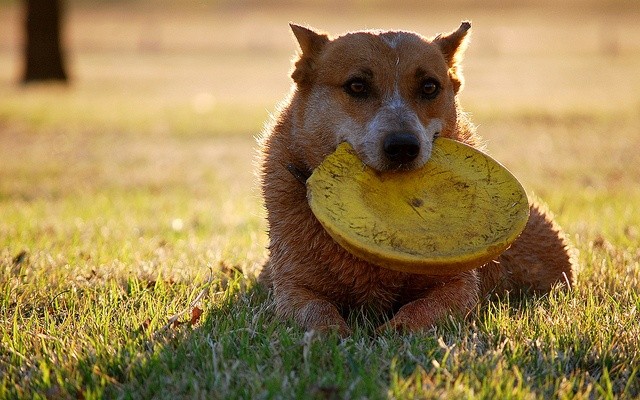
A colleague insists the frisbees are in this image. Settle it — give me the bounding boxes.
[306,134,531,275]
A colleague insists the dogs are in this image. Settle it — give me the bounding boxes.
[251,18,576,347]
